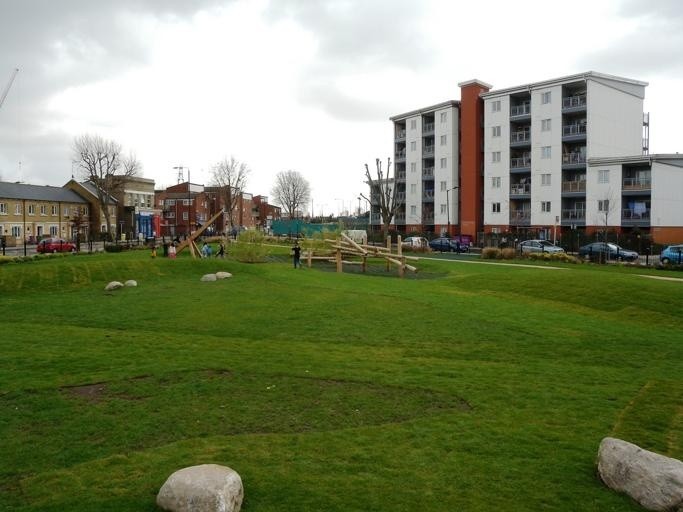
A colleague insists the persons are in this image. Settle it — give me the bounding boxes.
[162,231,191,266]
[455,240,460,255]
[196,228,239,260]
[150,247,157,258]
[291,244,302,270]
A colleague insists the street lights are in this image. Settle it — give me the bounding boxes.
[447,186,461,238]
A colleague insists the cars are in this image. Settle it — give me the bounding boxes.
[660,244,683,264]
[579,241,639,262]
[517,240,564,254]
[401,237,469,254]
[37,238,77,253]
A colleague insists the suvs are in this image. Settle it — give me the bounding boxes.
[228,225,247,236]
